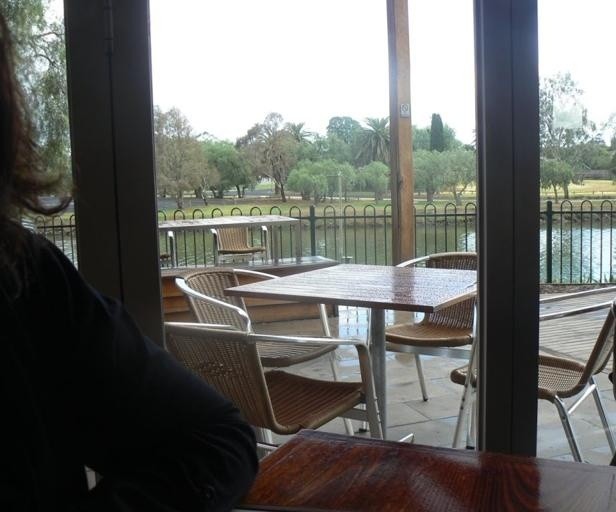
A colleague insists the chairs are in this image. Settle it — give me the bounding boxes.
[174,267,355,435]
[163,322,384,459]
[158,226,269,266]
[368,253,478,402]
[450,286,616,463]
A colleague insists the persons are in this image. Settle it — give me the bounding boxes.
[0,4,260,512]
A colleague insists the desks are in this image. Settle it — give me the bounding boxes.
[234,426,614,510]
[157,215,302,267]
